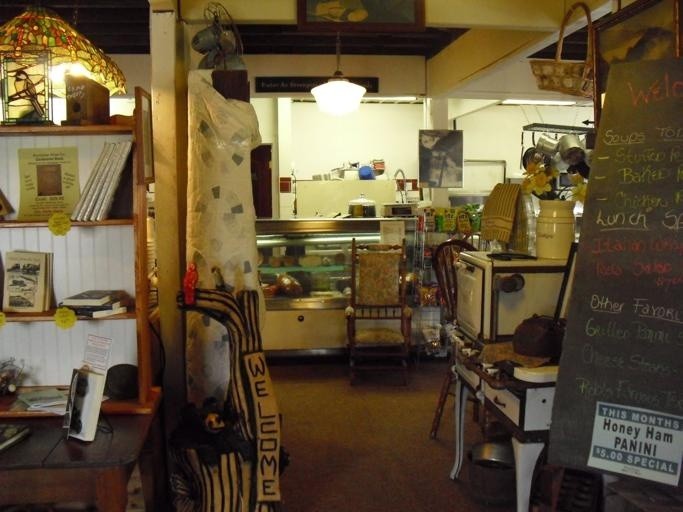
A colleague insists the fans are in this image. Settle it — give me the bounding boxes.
[190,1,244,69]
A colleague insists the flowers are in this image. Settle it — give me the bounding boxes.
[520,152,590,206]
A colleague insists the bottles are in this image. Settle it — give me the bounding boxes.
[279,177,291,194]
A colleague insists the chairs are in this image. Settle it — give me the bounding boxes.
[430,238,480,440]
[345,238,414,388]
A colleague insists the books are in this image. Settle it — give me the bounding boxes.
[11,396,70,416]
[3,138,140,320]
[15,386,68,408]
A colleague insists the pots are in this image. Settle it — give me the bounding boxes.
[347,193,376,219]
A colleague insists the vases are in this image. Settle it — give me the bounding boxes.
[534,200,574,260]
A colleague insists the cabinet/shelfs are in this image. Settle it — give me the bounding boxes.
[255,217,425,359]
[0,85,160,415]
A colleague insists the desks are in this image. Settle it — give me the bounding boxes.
[0,414,165,512]
[445,324,608,512]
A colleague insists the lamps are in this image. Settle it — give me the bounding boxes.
[311,32,366,117]
[0,0,126,98]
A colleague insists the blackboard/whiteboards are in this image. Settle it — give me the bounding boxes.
[545,58,681,493]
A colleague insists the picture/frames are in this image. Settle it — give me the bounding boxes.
[0,51,52,126]
[134,85,156,186]
[296,0,426,34]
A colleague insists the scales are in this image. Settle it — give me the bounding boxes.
[380,201,421,217]
